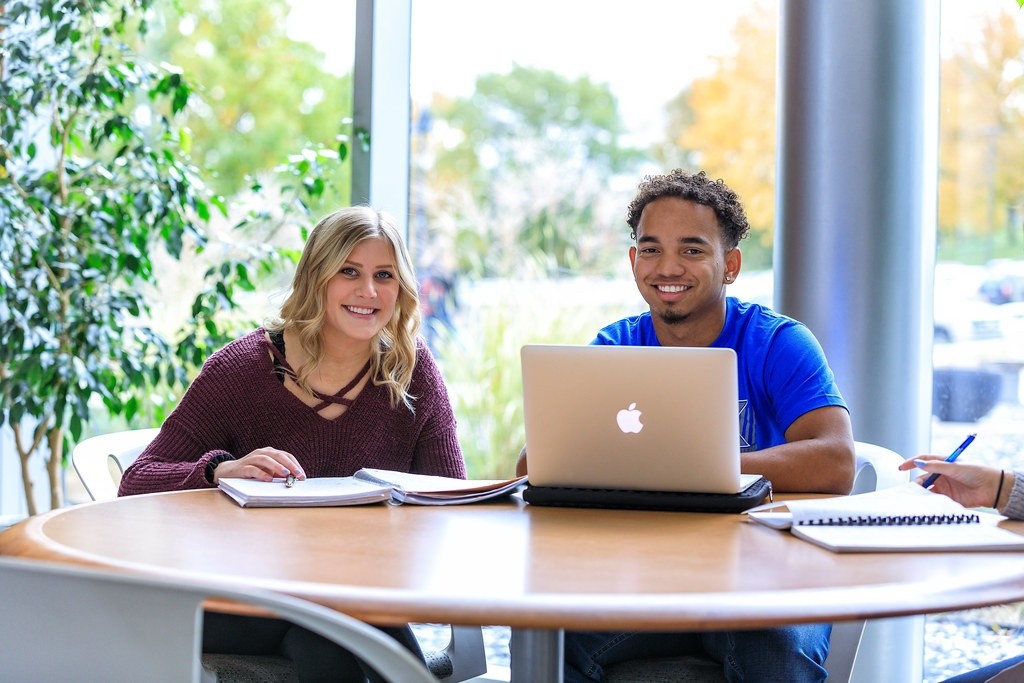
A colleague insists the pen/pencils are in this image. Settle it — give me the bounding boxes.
[286,474,296,487]
[920,430,977,488]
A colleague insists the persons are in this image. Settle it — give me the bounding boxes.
[901,453,1022,682]
[114,203,465,683]
[514,167,860,683]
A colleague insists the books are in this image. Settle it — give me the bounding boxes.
[218,468,529,511]
[742,477,1020,551]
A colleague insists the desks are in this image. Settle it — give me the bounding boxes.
[0,491,1024,683]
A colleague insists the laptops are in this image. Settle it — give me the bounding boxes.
[520,343,764,495]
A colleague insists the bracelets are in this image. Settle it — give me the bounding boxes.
[993,470,1005,509]
[205,451,235,483]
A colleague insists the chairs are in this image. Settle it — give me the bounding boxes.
[0,556,444,683]
[72,428,486,683]
[807,435,908,683]
